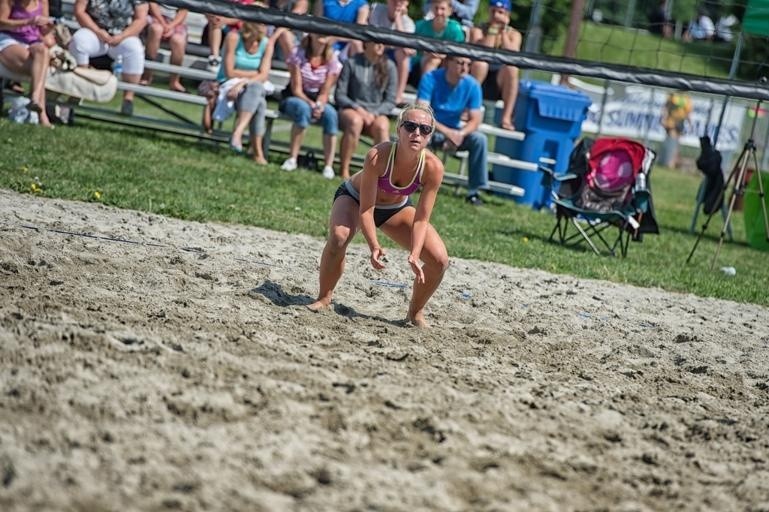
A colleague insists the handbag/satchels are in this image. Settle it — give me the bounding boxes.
[573,176,629,215]
[49,50,76,75]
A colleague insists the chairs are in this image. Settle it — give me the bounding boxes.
[547,136,656,259]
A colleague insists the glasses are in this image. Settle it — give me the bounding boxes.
[453,58,472,66]
[400,120,432,135]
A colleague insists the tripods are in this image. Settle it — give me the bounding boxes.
[687,100,769,272]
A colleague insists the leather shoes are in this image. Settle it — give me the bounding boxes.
[228,136,242,153]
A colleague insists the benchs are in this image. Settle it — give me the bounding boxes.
[0,0,537,198]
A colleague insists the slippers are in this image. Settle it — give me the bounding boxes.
[500,120,515,132]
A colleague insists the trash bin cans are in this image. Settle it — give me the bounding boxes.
[485,79,593,211]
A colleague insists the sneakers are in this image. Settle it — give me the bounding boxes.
[122,100,134,116]
[322,165,335,180]
[465,193,483,206]
[206,54,222,74]
[280,158,297,171]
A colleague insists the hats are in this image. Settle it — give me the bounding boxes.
[489,1,511,10]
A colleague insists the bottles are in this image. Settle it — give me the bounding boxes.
[113,56,124,80]
[635,172,646,190]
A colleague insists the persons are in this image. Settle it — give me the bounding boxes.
[197,0,523,206]
[0,0,190,127]
[583,1,740,50]
[303,105,449,327]
